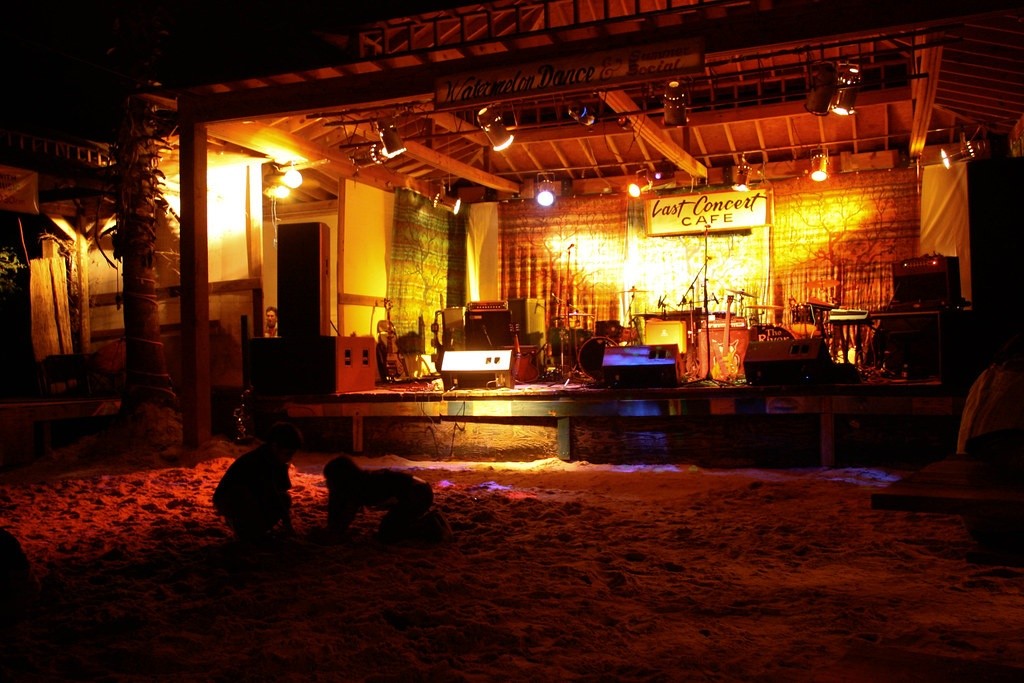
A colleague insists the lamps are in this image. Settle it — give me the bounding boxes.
[840,151,857,174]
[349,125,407,169]
[478,101,514,150]
[569,99,595,126]
[808,147,830,182]
[653,160,674,180]
[661,79,691,132]
[561,176,573,198]
[940,139,983,169]
[537,173,556,207]
[627,168,654,197]
[803,62,863,117]
[263,170,303,199]
[732,153,751,191]
[433,192,461,215]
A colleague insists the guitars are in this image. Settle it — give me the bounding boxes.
[384,298,405,378]
[711,295,738,381]
[509,321,539,383]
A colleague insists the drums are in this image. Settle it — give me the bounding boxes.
[750,304,818,341]
[548,320,638,383]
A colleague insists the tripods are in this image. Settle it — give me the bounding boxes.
[680,225,736,387]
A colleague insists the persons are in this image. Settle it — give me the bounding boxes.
[264,307,278,336]
[323,455,455,544]
[213,423,304,548]
[955,332,1024,454]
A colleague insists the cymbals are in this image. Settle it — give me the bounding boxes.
[725,289,758,298]
[744,305,785,310]
[804,280,840,288]
[558,311,593,316]
[619,289,650,293]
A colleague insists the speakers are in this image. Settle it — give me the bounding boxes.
[247,336,376,395]
[441,350,515,391]
[509,298,547,366]
[277,222,331,337]
[602,344,684,388]
[743,338,832,386]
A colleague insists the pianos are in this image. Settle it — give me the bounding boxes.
[829,308,869,367]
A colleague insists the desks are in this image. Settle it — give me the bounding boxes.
[872,462,1024,546]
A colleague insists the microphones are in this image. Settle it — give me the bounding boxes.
[567,243,574,249]
